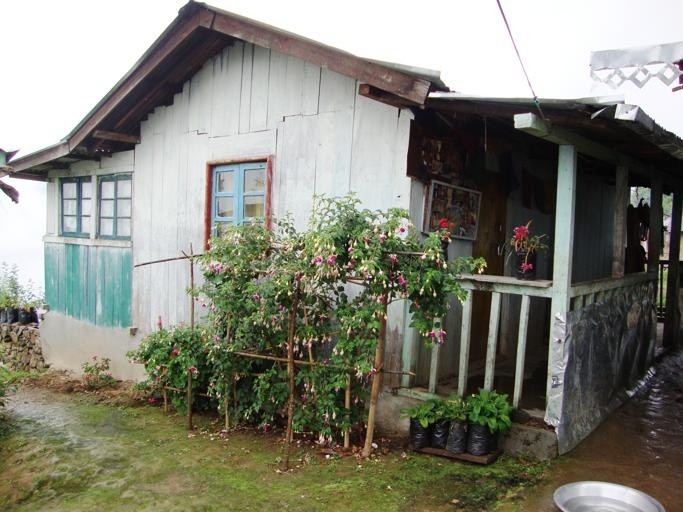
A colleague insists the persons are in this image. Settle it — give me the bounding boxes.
[624,232,647,274]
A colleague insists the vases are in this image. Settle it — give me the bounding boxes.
[517,266,537,280]
[410,416,489,454]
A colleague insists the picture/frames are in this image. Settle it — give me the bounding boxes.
[420,180,483,241]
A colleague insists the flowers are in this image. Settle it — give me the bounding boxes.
[498,217,552,278]
[400,387,515,436]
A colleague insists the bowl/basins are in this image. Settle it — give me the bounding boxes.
[553,480,665,512]
